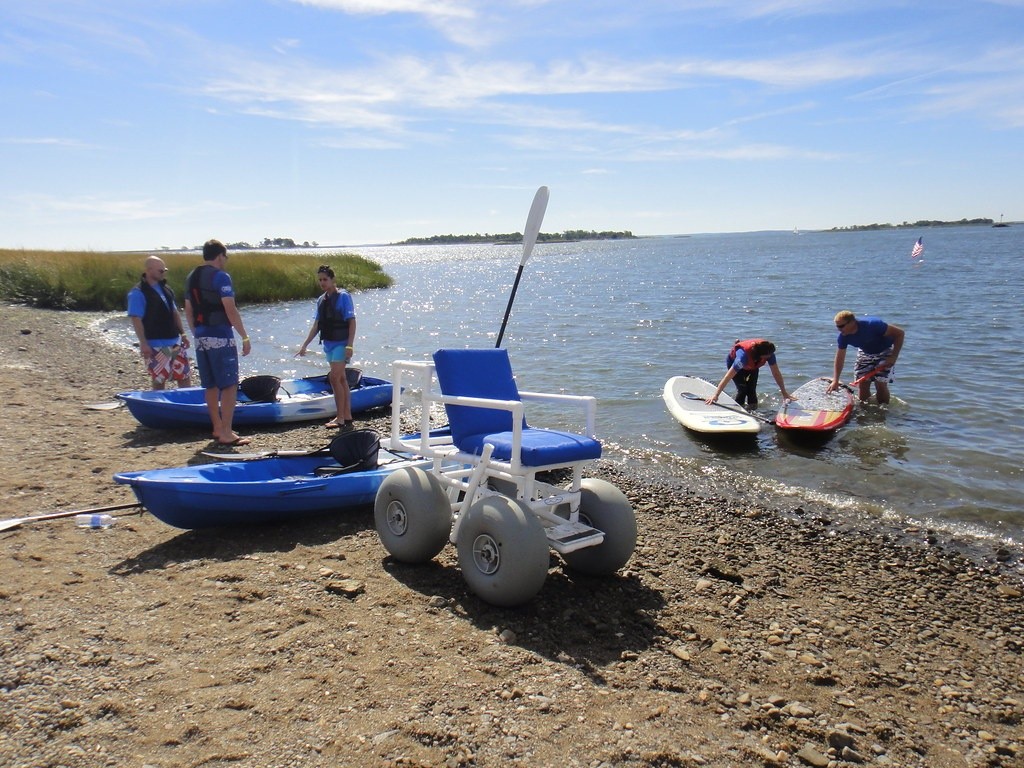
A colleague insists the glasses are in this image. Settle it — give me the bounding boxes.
[318,278,330,282]
[154,267,169,274]
[223,253,229,261]
[836,320,852,329]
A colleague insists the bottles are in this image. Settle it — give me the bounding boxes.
[74,515,117,527]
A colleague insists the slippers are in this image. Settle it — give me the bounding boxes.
[212,428,249,447]
[325,418,354,429]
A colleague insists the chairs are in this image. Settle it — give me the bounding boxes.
[432,347,602,469]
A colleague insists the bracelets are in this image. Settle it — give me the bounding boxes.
[345,345,353,349]
[182,333,186,337]
[242,335,249,341]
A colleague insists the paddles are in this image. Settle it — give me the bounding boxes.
[681,393,776,425]
[0,501,143,530]
[82,401,126,411]
[495,185,550,349]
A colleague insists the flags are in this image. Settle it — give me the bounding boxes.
[912,238,921,256]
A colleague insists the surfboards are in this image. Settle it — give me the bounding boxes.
[663,375,761,433]
[775,377,853,431]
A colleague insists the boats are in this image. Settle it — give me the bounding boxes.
[112,424,478,529]
[118,374,405,430]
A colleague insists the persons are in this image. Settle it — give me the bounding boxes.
[293,266,355,427]
[704,339,798,404]
[825,310,905,403]
[126,256,191,390]
[183,240,251,445]
